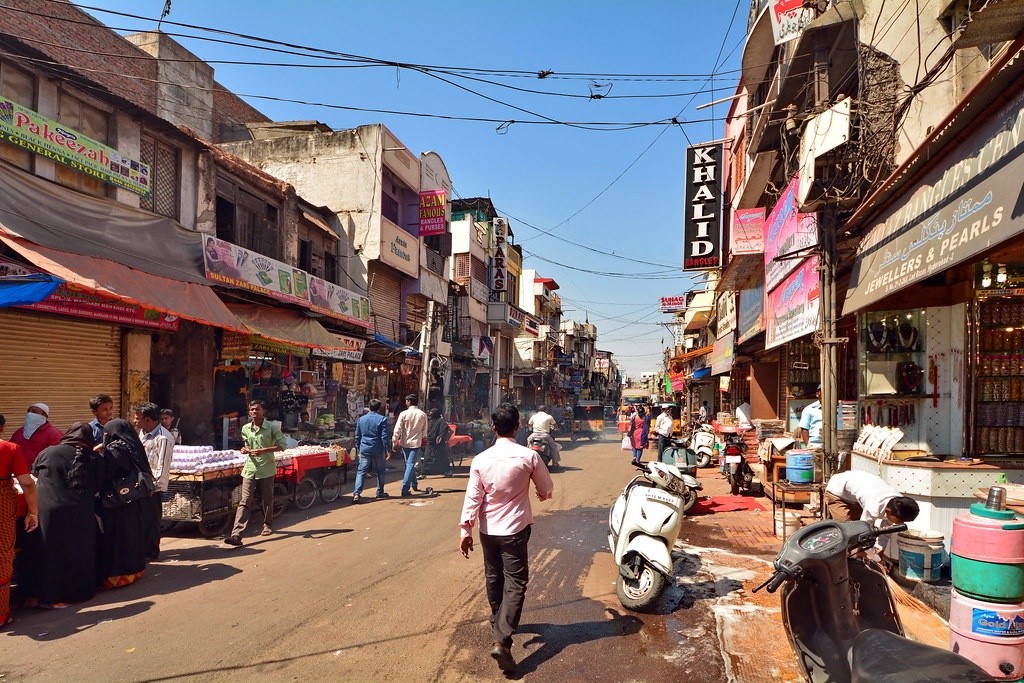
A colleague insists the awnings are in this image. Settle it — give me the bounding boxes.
[222,292,356,350]
[670,346,714,360]
[0,233,251,343]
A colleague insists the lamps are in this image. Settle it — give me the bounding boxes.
[374,366,378,372]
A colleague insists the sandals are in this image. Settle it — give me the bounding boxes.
[0,615,15,631]
[262,526,273,536]
[224,536,243,546]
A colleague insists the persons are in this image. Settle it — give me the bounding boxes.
[824,470,920,572]
[353,394,454,501]
[224,400,287,546]
[516,401,573,468]
[626,397,752,469]
[0,393,182,624]
[458,403,553,672]
[799,383,823,448]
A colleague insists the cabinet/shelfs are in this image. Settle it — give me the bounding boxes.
[866,310,934,400]
[788,342,841,398]
[970,266,1024,456]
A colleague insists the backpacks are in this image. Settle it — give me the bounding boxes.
[65,446,95,502]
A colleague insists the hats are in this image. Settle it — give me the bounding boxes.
[661,405,669,408]
[31,403,50,416]
[817,383,821,389]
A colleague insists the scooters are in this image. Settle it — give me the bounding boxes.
[751,521,998,683]
[607,455,706,612]
[644,413,756,515]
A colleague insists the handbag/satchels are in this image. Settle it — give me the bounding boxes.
[259,350,273,382]
[622,435,632,451]
[99,445,139,508]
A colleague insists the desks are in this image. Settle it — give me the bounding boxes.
[397,426,472,470]
[771,481,825,545]
[165,459,296,536]
[763,456,811,503]
[273,446,349,507]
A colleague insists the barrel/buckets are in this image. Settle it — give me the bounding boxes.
[786,453,813,484]
[774,512,801,542]
[474,440,484,452]
[896,530,945,582]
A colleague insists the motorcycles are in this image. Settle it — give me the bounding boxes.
[526,422,560,468]
[552,388,683,444]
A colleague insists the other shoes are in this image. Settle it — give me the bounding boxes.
[418,475,425,480]
[551,463,564,472]
[402,492,417,498]
[413,487,421,491]
[445,472,452,478]
[52,602,78,609]
[491,641,516,671]
[352,495,360,504]
[375,493,389,500]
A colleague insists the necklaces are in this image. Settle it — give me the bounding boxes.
[897,325,915,348]
[868,324,888,349]
